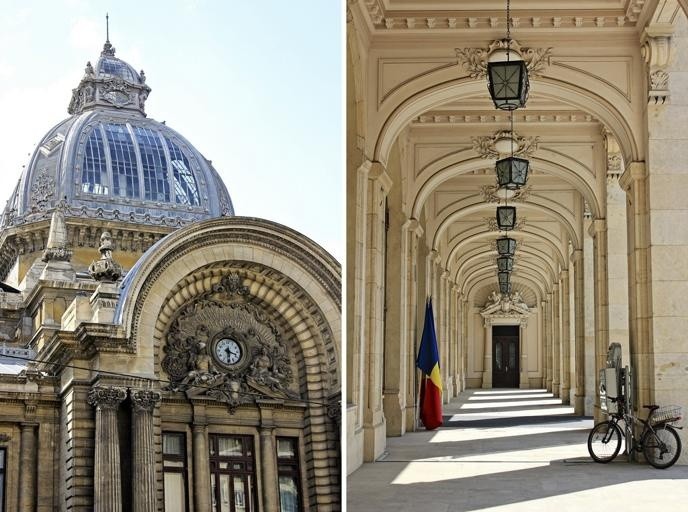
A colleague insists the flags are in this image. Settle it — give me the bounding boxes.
[415,294,444,432]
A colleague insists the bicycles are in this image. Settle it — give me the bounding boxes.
[586,394,684,470]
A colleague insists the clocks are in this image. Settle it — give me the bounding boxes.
[210,331,247,372]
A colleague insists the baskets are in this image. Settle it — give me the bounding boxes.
[651,405,682,424]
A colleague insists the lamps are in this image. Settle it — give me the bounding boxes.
[495,110,529,190]
[485,0,529,111]
[495,190,516,294]
